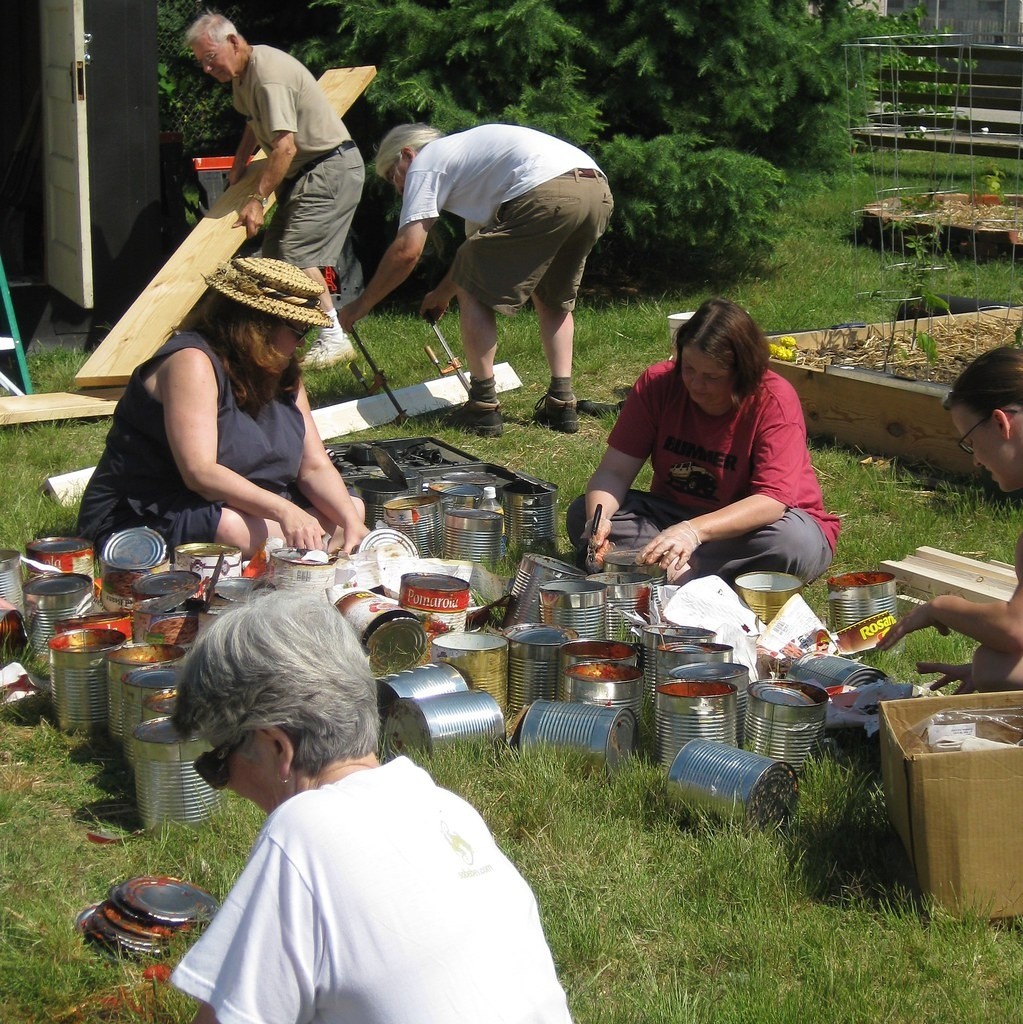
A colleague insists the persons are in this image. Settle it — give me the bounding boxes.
[171,587,574,1024]
[339,124,615,435]
[566,296,841,587]
[874,342,1023,696]
[184,7,365,371]
[75,258,371,563]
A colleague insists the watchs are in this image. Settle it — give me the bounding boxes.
[249,194,267,208]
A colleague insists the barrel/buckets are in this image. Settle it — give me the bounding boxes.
[0,478,898,838]
[667,311,696,344]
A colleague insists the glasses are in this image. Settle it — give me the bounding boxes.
[191,736,245,790]
[958,409,1018,454]
[194,42,226,68]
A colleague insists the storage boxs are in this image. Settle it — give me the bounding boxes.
[876,690,1023,926]
[349,462,535,504]
[326,431,485,492]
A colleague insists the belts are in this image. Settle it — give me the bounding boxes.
[282,139,356,185]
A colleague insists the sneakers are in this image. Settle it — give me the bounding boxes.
[535,393,579,433]
[447,398,503,435]
[301,334,356,369]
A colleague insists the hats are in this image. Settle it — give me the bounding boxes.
[206,257,335,328]
